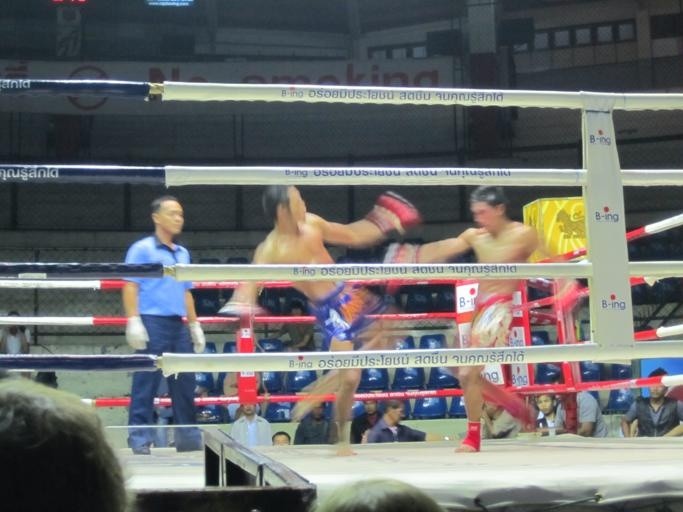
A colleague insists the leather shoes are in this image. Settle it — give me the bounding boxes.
[134,444,149,454]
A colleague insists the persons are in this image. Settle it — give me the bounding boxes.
[0,377,125,512]
[621,365,682,438]
[151,375,207,449]
[382,185,540,453]
[221,373,456,447]
[217,183,417,457]
[266,300,316,352]
[312,479,447,512]
[121,195,205,455]
[1,311,31,379]
[480,373,608,440]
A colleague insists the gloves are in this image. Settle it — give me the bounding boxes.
[189,322,206,353]
[364,192,419,232]
[126,316,149,350]
[384,239,420,263]
